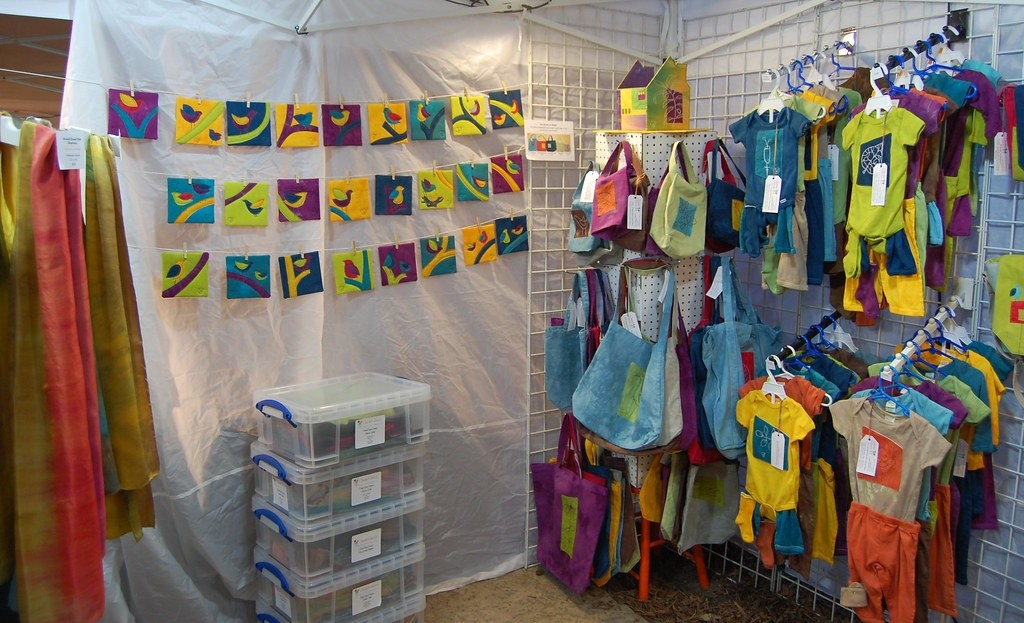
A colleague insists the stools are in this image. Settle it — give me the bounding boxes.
[630,487,709,602]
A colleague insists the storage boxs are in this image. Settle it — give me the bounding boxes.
[615,57,690,131]
[249,369,433,623]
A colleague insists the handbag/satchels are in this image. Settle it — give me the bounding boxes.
[516,134,787,598]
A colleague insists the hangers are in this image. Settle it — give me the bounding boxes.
[756,24,968,118]
[761,295,973,419]
